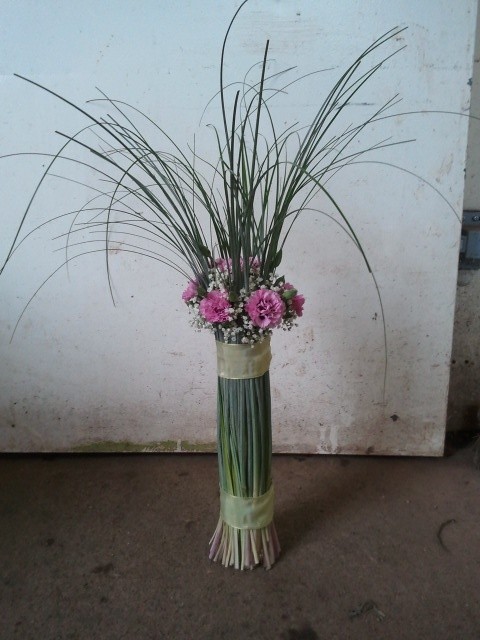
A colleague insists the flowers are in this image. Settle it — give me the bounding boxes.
[0,0,480,573]
